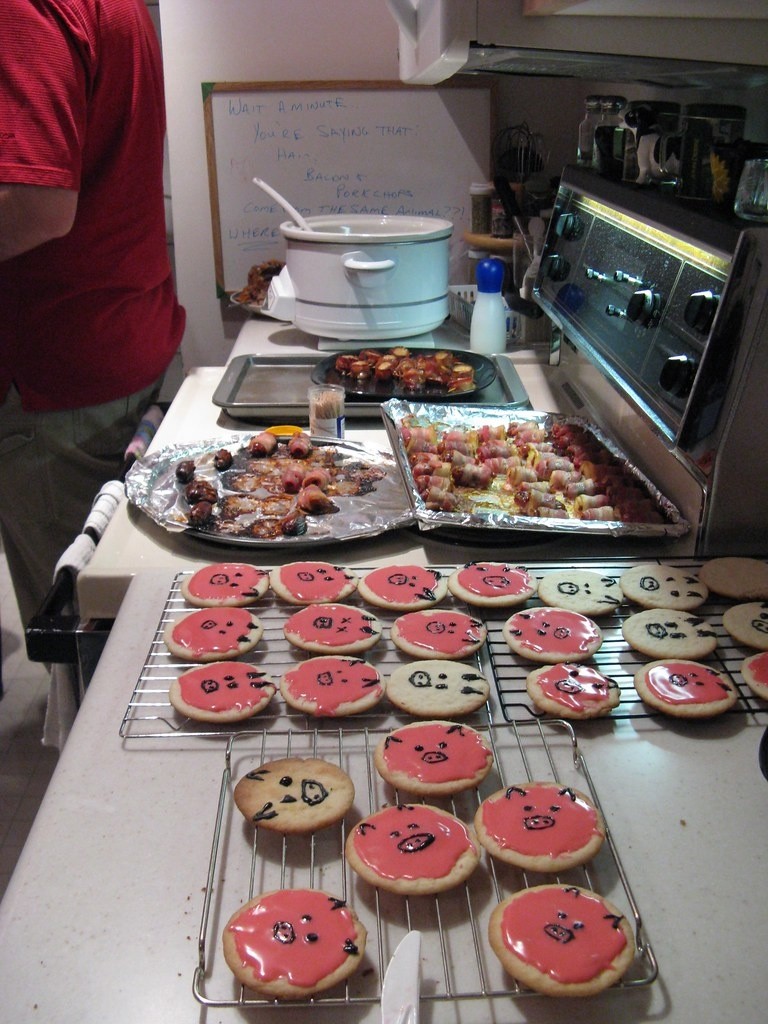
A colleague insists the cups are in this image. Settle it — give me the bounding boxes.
[623,100,768,223]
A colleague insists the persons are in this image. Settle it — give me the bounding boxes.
[1,0,187,676]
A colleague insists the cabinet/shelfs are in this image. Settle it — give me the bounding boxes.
[393,0,768,86]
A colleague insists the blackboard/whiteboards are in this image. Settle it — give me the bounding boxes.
[201,75,502,300]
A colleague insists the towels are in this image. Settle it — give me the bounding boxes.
[40,531,104,754]
[124,402,163,467]
[80,478,126,536]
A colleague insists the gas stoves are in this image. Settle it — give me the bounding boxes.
[74,166,768,623]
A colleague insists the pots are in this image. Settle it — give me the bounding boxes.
[279,213,454,342]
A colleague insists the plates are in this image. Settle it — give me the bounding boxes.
[311,347,497,401]
[230,290,262,314]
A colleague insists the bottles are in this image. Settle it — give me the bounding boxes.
[465,179,524,238]
[467,248,495,284]
[489,253,513,290]
[575,94,628,174]
[307,384,346,439]
[469,258,507,355]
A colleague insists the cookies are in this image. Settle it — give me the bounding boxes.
[164,556,768,997]
